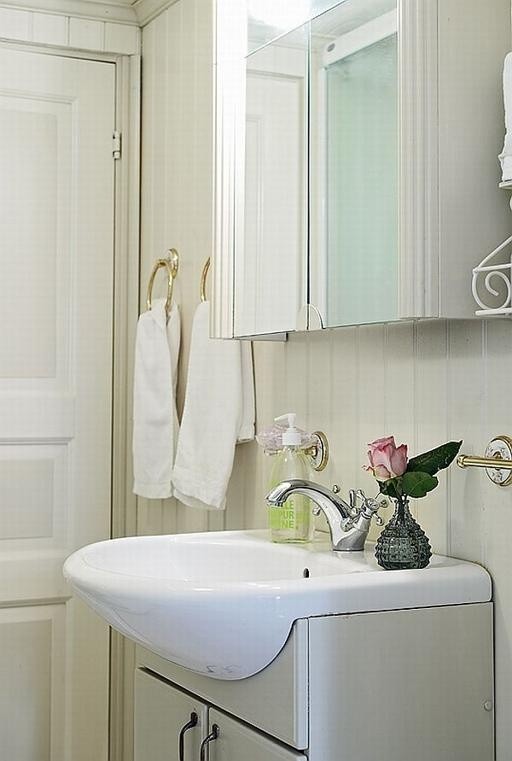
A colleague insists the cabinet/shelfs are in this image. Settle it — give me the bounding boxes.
[208,0,503,342]
[130,601,497,759]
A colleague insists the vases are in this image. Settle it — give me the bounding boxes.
[375,500,433,571]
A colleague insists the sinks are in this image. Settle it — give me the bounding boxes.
[82,537,368,587]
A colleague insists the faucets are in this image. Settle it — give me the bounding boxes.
[264,479,389,552]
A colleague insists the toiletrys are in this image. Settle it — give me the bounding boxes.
[266,411,312,544]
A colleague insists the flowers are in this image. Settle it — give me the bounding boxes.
[359,432,463,526]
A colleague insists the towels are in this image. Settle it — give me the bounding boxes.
[170,300,255,510]
[131,300,182,500]
